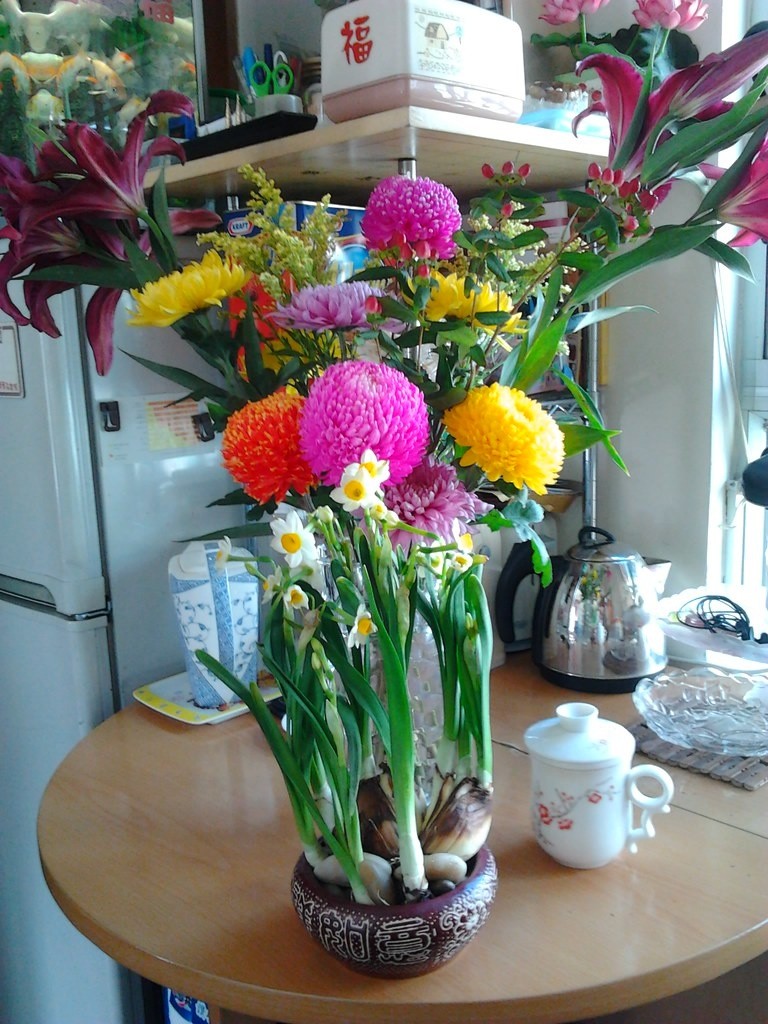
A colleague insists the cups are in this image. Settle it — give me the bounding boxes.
[166,547,257,705]
[524,702,672,869]
[255,95,304,118]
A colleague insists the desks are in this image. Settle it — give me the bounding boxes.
[35,643,768,1024]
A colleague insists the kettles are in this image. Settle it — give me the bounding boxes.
[495,528,672,693]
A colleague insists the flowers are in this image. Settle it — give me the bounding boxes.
[0,0,768,898]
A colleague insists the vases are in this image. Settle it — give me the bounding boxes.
[290,834,498,980]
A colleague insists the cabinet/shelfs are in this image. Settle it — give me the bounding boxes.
[141,106,610,673]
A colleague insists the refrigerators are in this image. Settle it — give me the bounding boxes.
[0,217,275,1024]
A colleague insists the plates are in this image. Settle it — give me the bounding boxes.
[133,663,281,726]
[634,669,768,756]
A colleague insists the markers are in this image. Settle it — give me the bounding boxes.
[232,42,303,98]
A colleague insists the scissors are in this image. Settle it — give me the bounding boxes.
[250,51,294,98]
